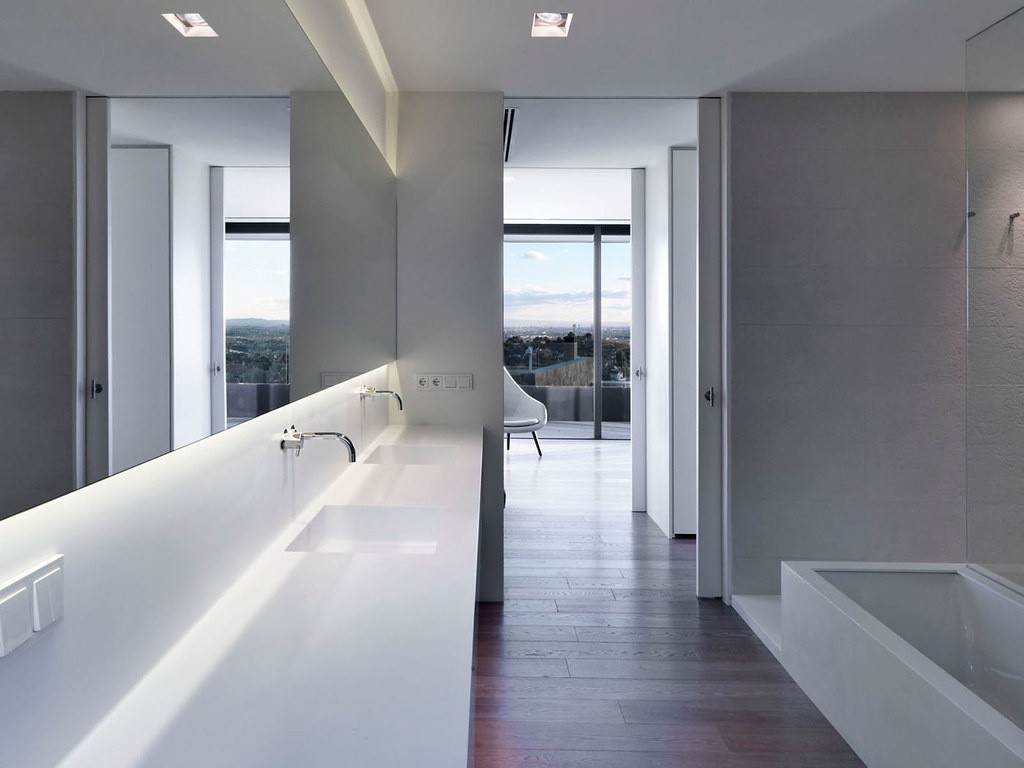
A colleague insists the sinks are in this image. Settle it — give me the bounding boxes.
[286,506,446,554]
[363,445,458,465]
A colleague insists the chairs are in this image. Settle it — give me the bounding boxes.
[503,367,548,456]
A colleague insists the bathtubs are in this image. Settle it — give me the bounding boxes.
[781,562,1023,768]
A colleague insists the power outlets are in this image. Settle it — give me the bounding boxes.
[429,374,443,389]
[415,374,429,389]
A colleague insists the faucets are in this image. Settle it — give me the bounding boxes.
[376,391,403,410]
[295,432,356,464]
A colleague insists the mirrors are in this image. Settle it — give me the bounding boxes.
[964,8,1024,600]
[0,0,397,520]
